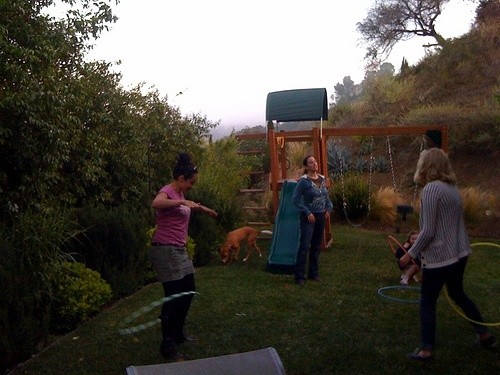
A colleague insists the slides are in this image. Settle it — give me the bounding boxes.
[266,180,303,274]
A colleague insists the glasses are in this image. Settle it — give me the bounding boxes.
[192,167,197,174]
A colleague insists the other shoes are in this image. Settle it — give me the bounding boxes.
[400,273,409,285]
[160,332,188,360]
[476,332,496,346]
[407,348,432,360]
[313,276,322,282]
[297,279,304,285]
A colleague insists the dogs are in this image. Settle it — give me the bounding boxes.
[218,227,273,264]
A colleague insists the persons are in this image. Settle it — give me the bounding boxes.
[292,154,334,287]
[148,152,218,363]
[394,230,422,286]
[404,148,500,359]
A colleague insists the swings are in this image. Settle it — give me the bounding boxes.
[338,136,374,227]
[386,136,424,222]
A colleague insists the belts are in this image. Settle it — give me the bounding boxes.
[151,242,179,247]
